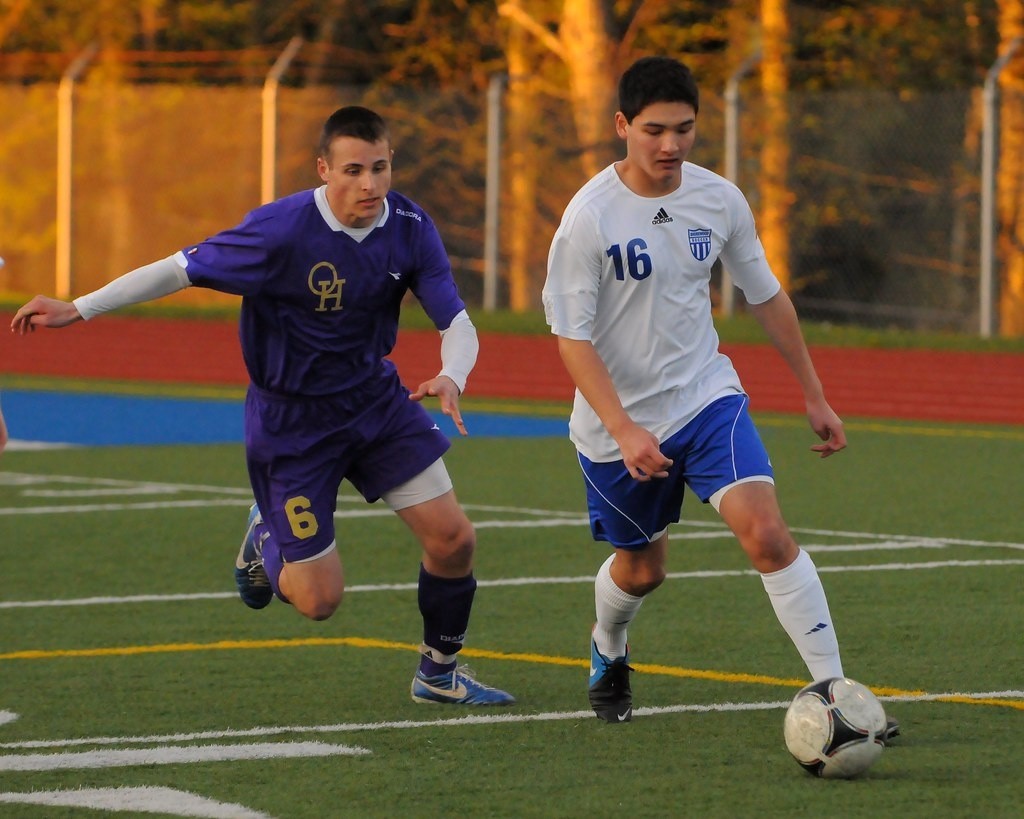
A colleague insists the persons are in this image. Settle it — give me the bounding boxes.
[543,55,902,737]
[11,103,514,705]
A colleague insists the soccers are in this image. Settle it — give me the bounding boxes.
[782,675,889,781]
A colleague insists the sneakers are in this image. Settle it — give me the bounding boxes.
[885,715,901,739]
[410,664,516,707]
[588,621,637,723]
[235,503,274,609]
